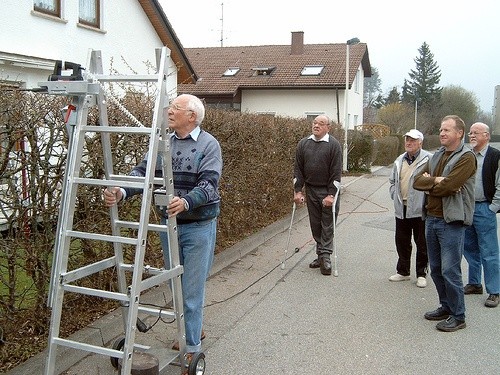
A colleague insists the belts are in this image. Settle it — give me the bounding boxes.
[403,200,408,205]
[176,218,210,225]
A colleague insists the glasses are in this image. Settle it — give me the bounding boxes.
[468,132,488,136]
[170,103,195,112]
[311,122,331,126]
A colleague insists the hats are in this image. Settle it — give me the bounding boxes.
[402,129,424,141]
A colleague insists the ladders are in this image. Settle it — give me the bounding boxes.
[43,49,206,375]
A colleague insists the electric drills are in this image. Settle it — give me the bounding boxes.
[16,59,87,93]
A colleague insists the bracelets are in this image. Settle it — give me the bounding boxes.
[182,199,188,211]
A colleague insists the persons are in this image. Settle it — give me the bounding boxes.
[292,115,342,275]
[412,115,479,332]
[459,122,500,308]
[103,94,222,366]
[388,129,433,288]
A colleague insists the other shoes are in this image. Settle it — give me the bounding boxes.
[181,352,195,375]
[416,277,427,287]
[485,294,500,307]
[389,274,412,282]
[320,258,332,275]
[172,326,206,351]
[424,306,450,321]
[309,257,321,268]
[436,316,467,332]
[462,284,483,294]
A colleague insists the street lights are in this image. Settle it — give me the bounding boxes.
[343,37,361,171]
[414,97,423,130]
